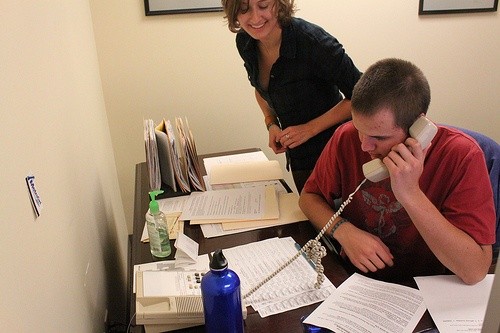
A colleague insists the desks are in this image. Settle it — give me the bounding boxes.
[126,147,436,333]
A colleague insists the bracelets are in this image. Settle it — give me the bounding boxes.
[266,121,280,131]
[329,218,350,240]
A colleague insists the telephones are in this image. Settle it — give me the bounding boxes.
[362,117,438,182]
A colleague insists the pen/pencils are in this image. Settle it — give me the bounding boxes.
[295,243,318,273]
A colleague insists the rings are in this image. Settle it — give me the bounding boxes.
[286,134,289,139]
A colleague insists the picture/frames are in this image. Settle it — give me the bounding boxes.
[418,0,499,15]
[144,0,226,17]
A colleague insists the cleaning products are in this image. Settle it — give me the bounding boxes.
[145,190,172,258]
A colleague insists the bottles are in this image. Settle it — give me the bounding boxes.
[200,249,246,333]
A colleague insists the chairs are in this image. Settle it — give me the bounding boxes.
[440,124,500,280]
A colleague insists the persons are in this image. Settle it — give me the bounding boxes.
[298,59,496,286]
[221,0,363,197]
